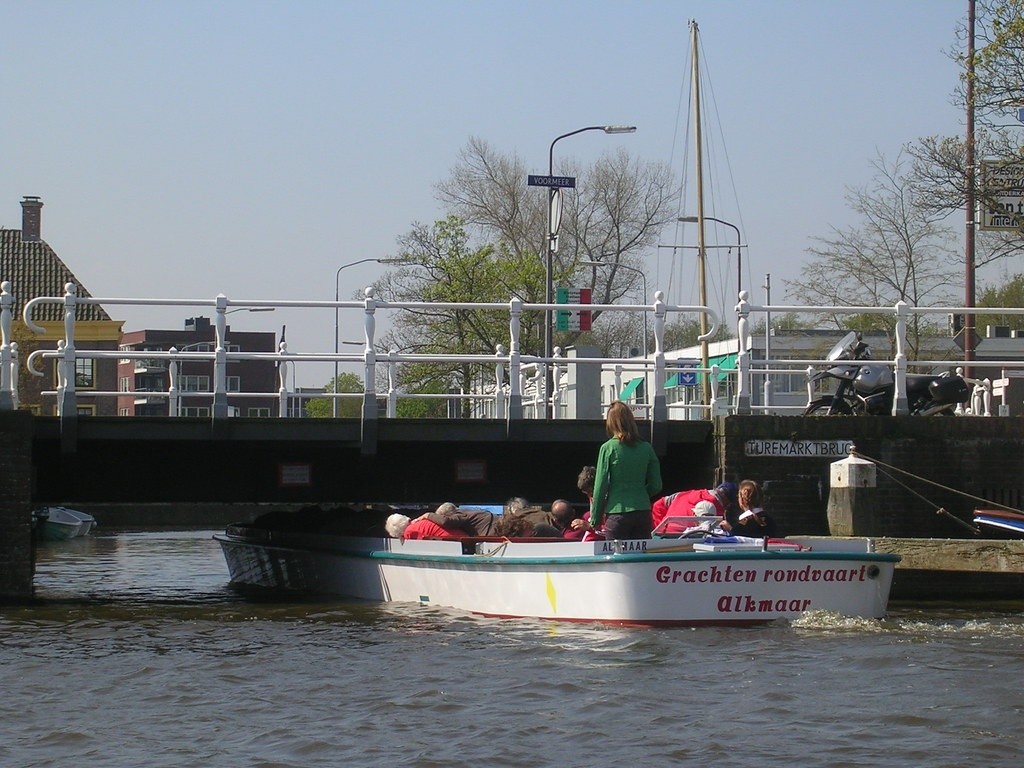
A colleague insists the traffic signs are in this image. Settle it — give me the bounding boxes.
[677,358,697,385]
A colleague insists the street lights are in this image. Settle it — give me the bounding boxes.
[676,214,743,354]
[177,339,230,417]
[332,258,393,418]
[543,123,637,419]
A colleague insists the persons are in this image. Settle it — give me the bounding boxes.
[650,478,773,538]
[589,400,663,540]
[383,465,609,542]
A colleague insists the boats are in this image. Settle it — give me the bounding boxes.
[57,507,95,537]
[212,518,903,632]
[973,507,1024,540]
[32,507,83,540]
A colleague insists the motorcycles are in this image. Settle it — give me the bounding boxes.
[801,330,969,417]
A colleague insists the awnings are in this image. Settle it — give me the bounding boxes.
[619,378,644,402]
[696,356,727,383]
[699,352,750,382]
[664,372,677,390]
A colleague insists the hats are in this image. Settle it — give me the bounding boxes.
[718,482,737,501]
